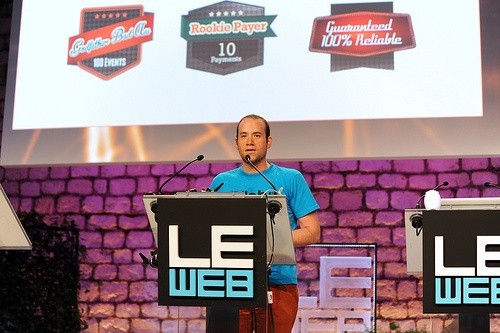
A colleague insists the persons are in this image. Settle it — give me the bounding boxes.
[206,113,320,333]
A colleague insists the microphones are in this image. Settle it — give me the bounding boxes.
[156,154,204,195]
[484,181,500,191]
[246,154,277,195]
[416,181,448,209]
